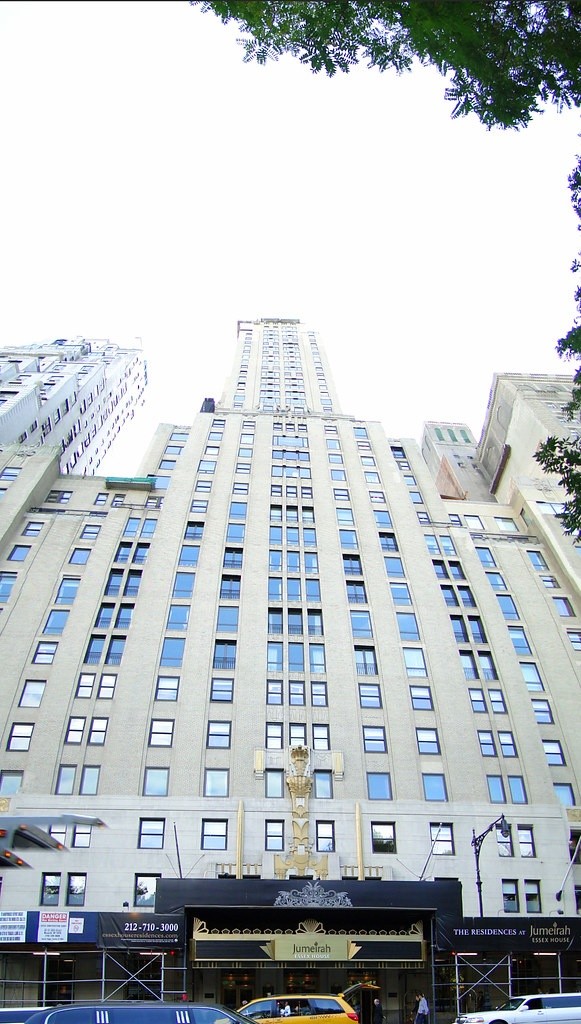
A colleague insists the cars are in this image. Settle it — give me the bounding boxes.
[0,999,264,1024]
[210,982,382,1024]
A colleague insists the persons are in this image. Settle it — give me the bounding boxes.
[537,988,542,994]
[352,997,360,1015]
[476,991,485,1007]
[280,1002,292,1017]
[242,1001,248,1014]
[411,993,430,1024]
[374,999,387,1024]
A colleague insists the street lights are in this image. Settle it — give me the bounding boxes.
[471,814,510,1011]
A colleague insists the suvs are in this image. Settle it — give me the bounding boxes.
[453,992,581,1024]
